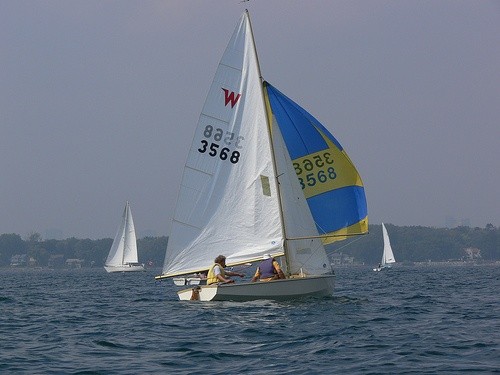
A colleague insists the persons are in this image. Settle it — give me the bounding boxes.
[207,255,246,285]
[250,254,286,283]
[378,263,382,268]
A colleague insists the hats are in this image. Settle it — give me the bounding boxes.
[261,253,274,260]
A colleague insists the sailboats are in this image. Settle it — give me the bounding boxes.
[153,9,370,302]
[373,222,396,272]
[104,200,147,273]
